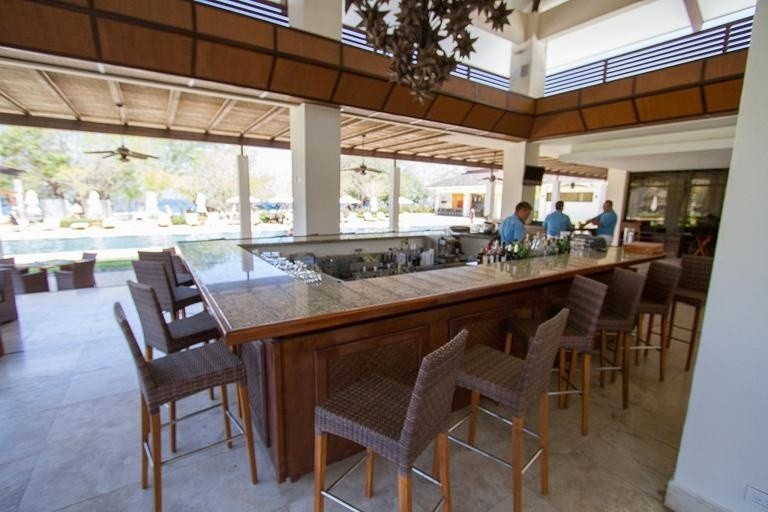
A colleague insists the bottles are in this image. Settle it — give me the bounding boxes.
[387,239,419,275]
[439,235,462,257]
[497,253,571,277]
[475,231,567,266]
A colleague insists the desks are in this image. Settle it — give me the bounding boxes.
[691,229,715,256]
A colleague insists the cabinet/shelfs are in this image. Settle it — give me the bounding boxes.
[617,220,650,244]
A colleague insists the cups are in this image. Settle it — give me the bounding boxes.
[623,227,636,245]
[259,251,323,288]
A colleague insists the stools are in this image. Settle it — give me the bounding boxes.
[645,256,713,373]
[314,327,471,509]
[430,306,570,511]
[611,259,685,382]
[495,272,610,437]
[567,266,647,410]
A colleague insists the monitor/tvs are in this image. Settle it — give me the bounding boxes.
[524,166,544,185]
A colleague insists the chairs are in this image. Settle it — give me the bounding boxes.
[113,251,258,512]
[1,253,98,325]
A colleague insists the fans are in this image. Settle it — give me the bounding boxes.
[84,147,159,162]
[560,179,588,192]
[478,175,502,183]
[342,165,381,175]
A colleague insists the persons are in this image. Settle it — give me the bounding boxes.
[543,201,573,238]
[499,202,533,244]
[587,200,617,246]
